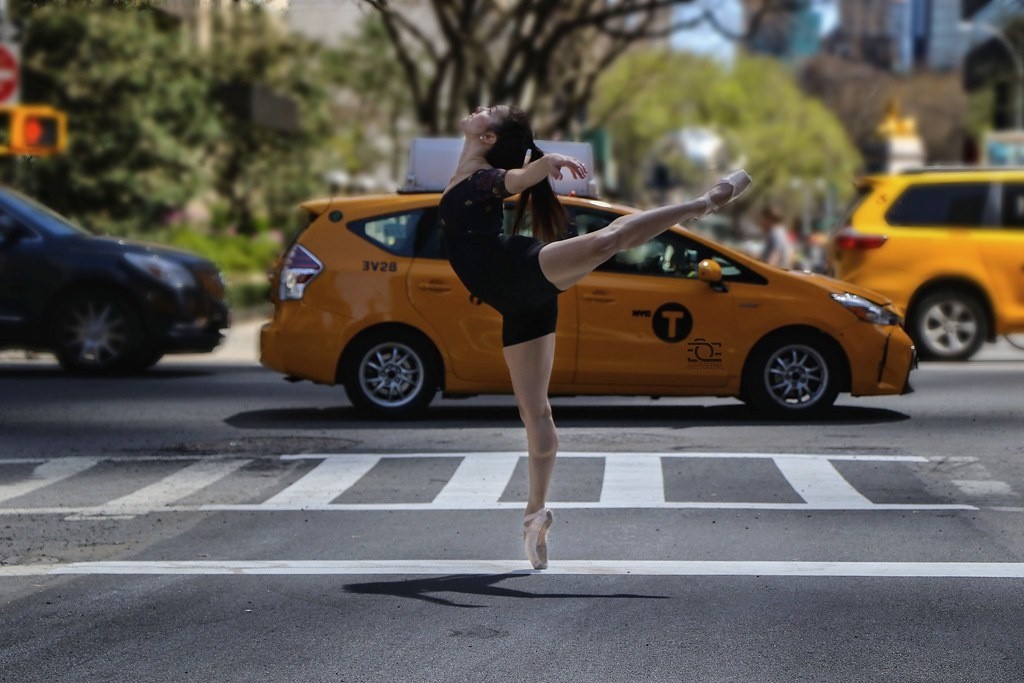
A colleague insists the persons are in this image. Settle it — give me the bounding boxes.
[754,203,843,275]
[435,102,751,571]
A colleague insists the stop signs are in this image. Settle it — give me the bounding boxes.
[0,39,23,108]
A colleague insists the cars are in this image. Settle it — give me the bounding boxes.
[0,183,230,379]
[259,135,920,422]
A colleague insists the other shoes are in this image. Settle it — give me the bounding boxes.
[521,508,553,569]
[695,169,752,220]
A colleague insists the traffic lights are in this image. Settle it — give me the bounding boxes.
[0,106,67,154]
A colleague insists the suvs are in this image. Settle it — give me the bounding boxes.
[827,168,1024,361]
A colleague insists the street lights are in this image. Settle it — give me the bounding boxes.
[953,20,1023,83]
